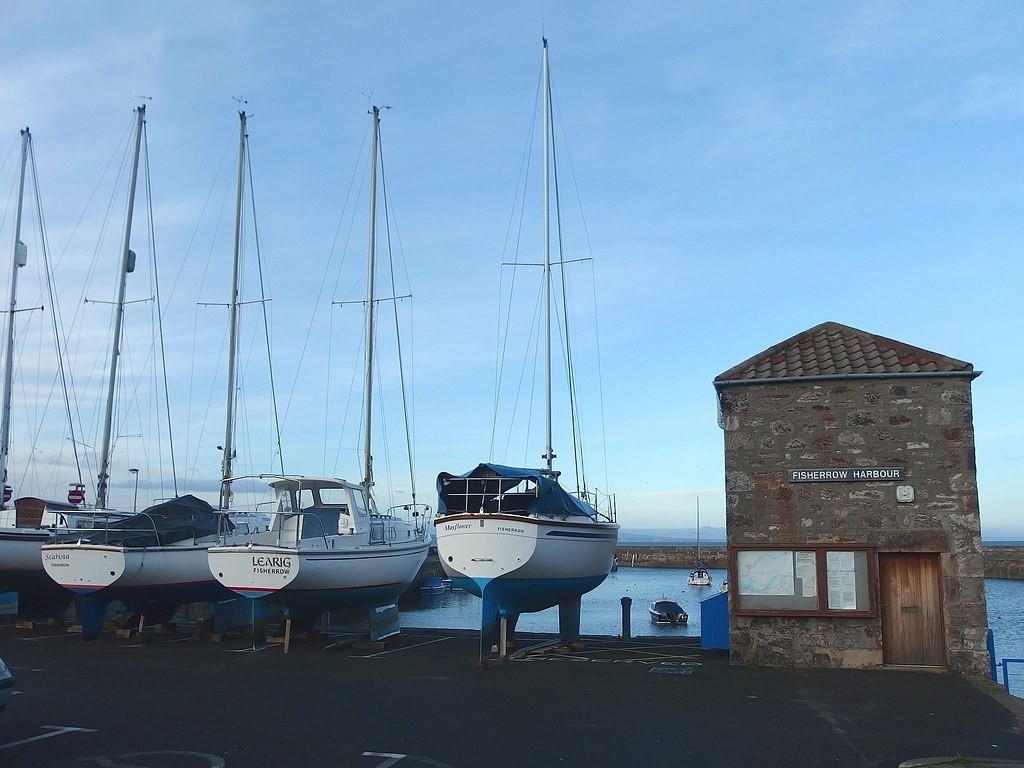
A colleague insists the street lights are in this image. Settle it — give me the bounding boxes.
[127,467,139,517]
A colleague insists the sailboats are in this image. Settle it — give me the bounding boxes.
[2,126,137,624]
[8,102,271,641]
[207,105,429,651]
[685,495,712,586]
[431,34,622,665]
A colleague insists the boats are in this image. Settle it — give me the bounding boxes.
[649,600,689,626]
[419,577,447,595]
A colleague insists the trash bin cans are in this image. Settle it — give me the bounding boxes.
[697,590,730,652]
